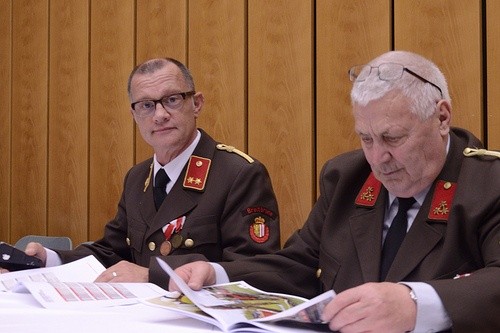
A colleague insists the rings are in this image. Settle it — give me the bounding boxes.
[112,271,117,277]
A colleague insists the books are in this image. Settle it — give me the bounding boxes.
[140,253,337,332]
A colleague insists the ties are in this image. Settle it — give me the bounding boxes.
[380,197,417,283]
[153,168,171,211]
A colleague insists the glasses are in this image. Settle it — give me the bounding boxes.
[131,90,196,112]
[348,62,445,99]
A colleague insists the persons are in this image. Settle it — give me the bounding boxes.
[167,50,499,333]
[0,56,281,287]
